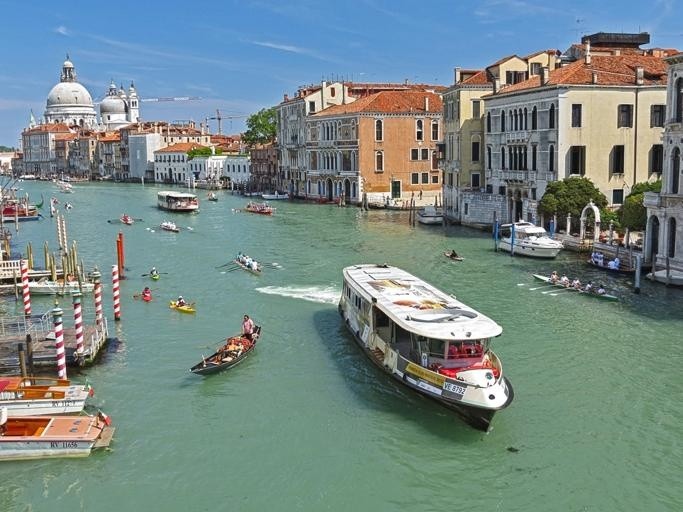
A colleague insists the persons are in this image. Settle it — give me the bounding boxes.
[150,267,157,274]
[142,286,151,297]
[549,251,621,295]
[175,295,185,307]
[241,314,255,342]
[451,250,458,258]
[235,251,258,272]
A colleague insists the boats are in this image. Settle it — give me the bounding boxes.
[442,250,464,262]
[339,264,514,433]
[416,208,444,225]
[120,213,180,233]
[141,269,198,315]
[498,220,622,301]
[157,190,199,212]
[233,257,262,276]
[189,325,266,376]
[244,194,289,215]
[0,376,114,463]
[14,281,95,296]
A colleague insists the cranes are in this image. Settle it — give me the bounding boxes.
[207,109,250,135]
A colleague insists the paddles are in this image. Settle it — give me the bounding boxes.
[176,225,192,231]
[140,272,168,277]
[145,226,164,232]
[218,261,284,275]
[516,281,579,296]
[133,287,159,299]
